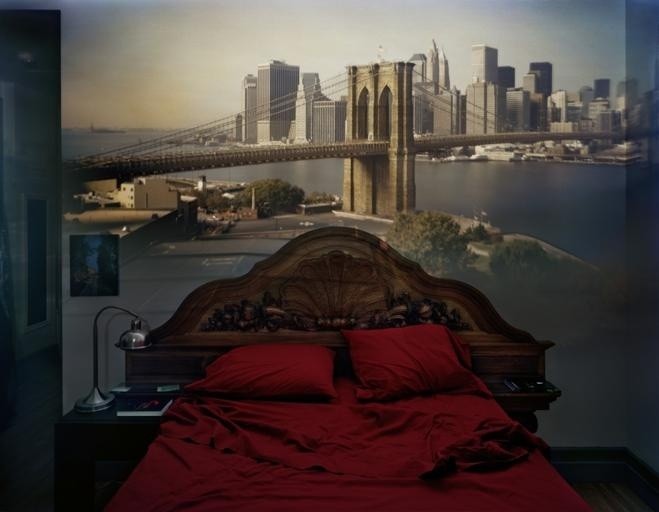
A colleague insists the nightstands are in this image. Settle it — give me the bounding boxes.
[54,392,174,512]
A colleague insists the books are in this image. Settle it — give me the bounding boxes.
[115,399,173,417]
[109,383,181,394]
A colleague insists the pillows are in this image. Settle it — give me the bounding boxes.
[338,321,474,403]
[183,324,340,402]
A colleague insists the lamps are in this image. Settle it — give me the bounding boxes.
[74,305,152,414]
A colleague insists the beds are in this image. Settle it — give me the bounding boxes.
[92,225,595,512]
